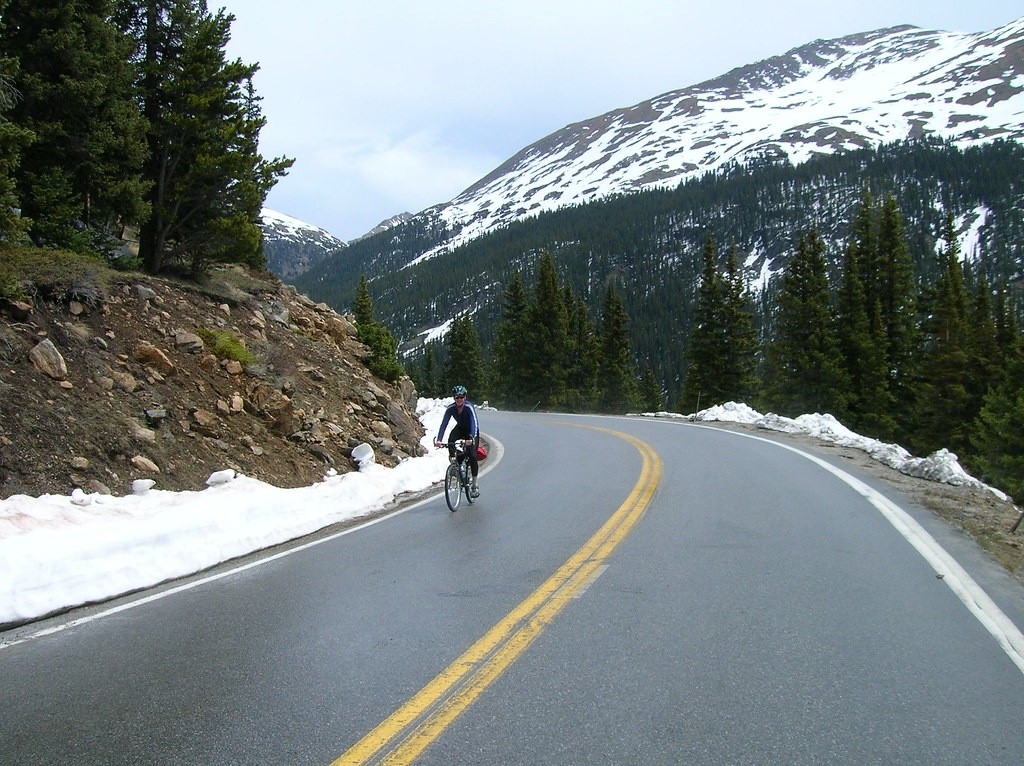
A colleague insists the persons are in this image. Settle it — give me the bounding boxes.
[436,385,479,498]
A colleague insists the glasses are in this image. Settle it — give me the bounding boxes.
[455,396,464,399]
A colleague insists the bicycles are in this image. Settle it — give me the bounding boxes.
[433,436,481,512]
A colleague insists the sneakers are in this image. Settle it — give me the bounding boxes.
[471,484,480,498]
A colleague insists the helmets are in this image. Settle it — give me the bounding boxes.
[451,386,467,395]
